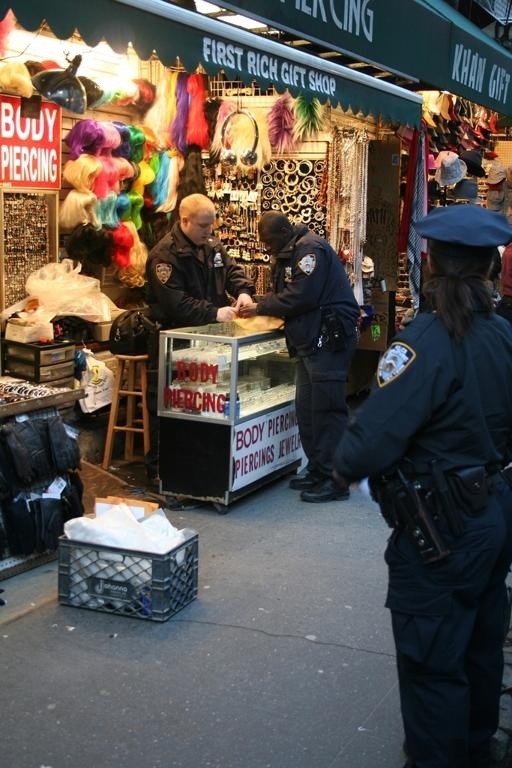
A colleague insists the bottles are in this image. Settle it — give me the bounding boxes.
[224,392,240,421]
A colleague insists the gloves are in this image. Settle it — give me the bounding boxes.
[0,415,91,559]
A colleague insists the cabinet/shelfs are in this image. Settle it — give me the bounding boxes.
[148,310,303,517]
[0,386,94,586]
[0,331,81,425]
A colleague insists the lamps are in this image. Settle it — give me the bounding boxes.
[26,48,91,118]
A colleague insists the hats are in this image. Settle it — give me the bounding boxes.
[415,202,512,248]
[415,90,512,203]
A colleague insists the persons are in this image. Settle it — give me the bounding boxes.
[331,203,512,767]
[145,194,254,495]
[494,243,511,322]
[239,211,358,502]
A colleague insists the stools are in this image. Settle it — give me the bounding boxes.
[103,349,154,474]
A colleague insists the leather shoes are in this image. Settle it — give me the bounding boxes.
[288,474,329,490]
[300,482,351,504]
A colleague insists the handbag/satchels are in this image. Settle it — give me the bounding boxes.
[110,306,161,356]
